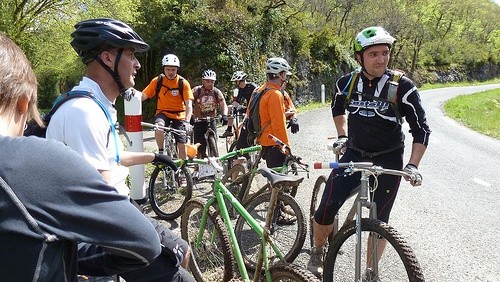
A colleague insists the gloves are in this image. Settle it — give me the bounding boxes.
[220,116,228,126]
[287,118,299,134]
[190,116,195,125]
[182,121,193,135]
[124,89,135,101]
[152,153,178,172]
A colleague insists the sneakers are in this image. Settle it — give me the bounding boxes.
[306,242,326,277]
[362,274,380,282]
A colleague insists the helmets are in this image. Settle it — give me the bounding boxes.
[162,54,180,67]
[230,71,248,81]
[354,26,392,54]
[202,70,216,81]
[265,57,292,74]
[70,19,149,63]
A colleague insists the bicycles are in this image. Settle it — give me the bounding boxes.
[310,141,427,282]
[140,105,321,282]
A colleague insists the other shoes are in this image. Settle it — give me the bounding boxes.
[219,131,232,138]
[277,211,297,223]
[283,206,296,215]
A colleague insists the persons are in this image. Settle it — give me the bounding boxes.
[307,26,431,282]
[275,68,300,135]
[244,57,303,224]
[186,69,229,169]
[36,17,200,282]
[124,53,195,183]
[1,28,161,282]
[219,70,259,143]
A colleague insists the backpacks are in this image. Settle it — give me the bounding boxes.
[247,87,283,137]
